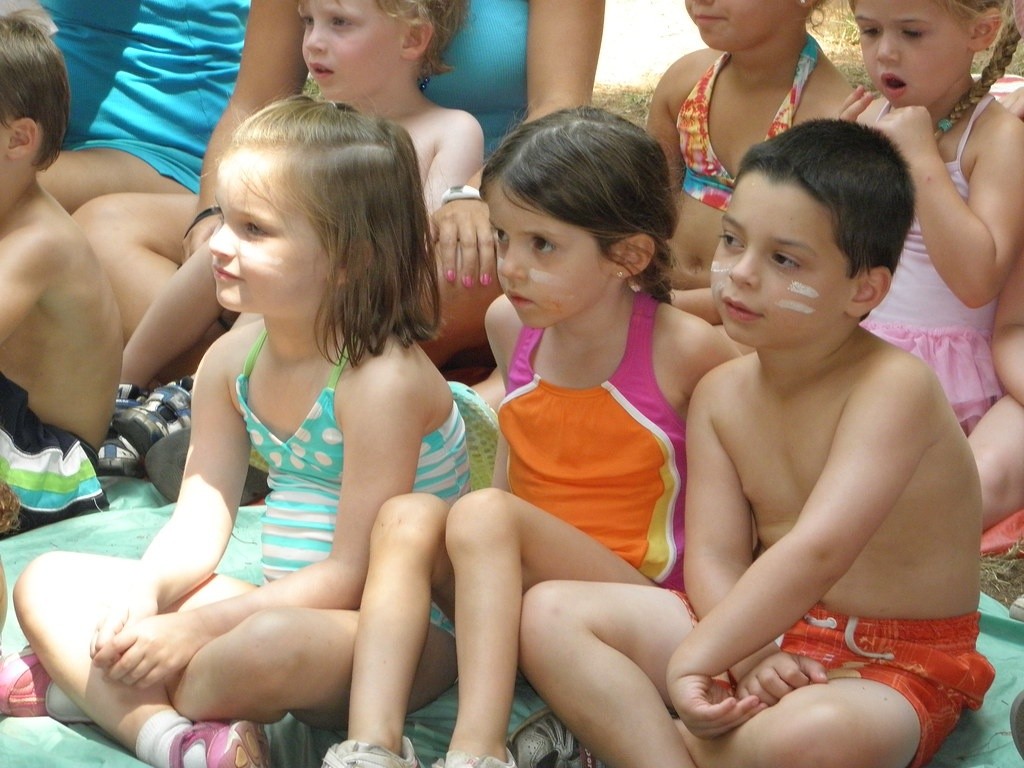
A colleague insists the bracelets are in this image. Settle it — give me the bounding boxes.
[440,184,484,204]
[184,206,222,238]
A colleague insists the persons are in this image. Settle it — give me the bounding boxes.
[0,0,1024,768]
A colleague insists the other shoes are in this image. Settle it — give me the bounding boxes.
[0,646,93,721]
[168,719,271,768]
[146,427,273,507]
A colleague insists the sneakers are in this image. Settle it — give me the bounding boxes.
[96,383,155,475]
[118,377,194,453]
[432,746,517,768]
[507,705,606,768]
[320,736,421,768]
